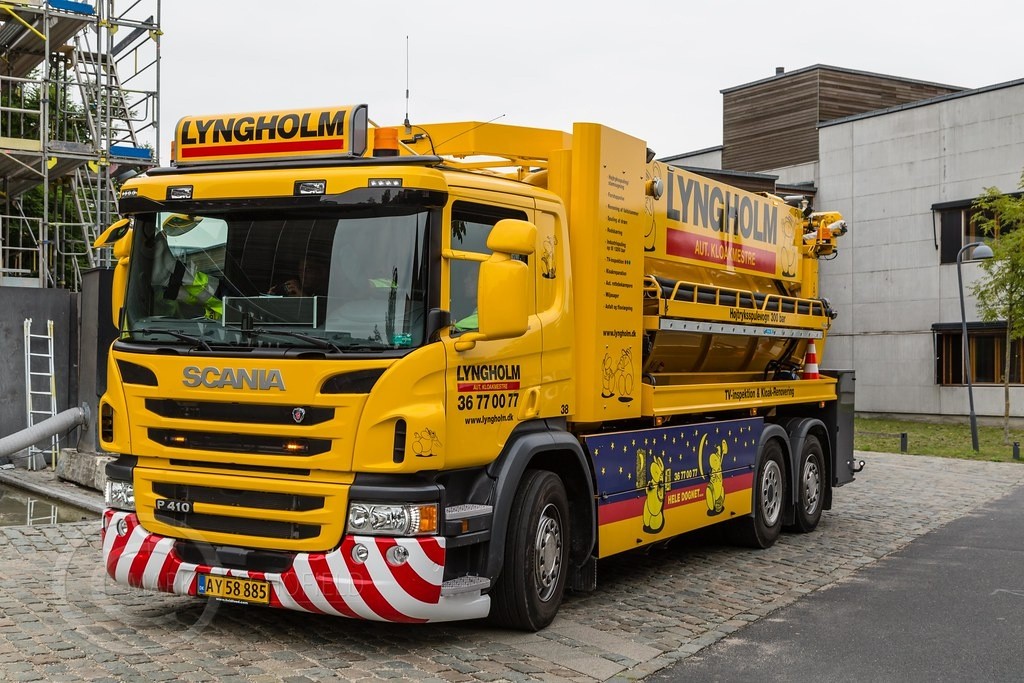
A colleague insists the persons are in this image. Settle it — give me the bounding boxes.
[450,265,478,333]
[266,254,327,324]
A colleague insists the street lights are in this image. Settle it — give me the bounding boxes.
[953,240,996,449]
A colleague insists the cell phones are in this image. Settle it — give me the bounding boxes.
[282,281,294,291]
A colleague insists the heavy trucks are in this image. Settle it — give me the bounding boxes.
[87,98,865,634]
